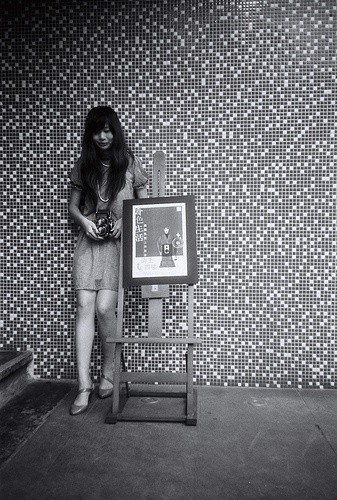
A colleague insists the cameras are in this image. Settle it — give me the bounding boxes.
[93,210,115,238]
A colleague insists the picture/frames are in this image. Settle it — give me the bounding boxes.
[123,195,197,285]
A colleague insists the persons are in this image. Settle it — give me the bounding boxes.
[68,106,147,415]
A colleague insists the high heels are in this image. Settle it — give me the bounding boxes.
[71,383,96,415]
[98,374,114,398]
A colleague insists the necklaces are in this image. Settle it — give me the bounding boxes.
[96,157,113,202]
